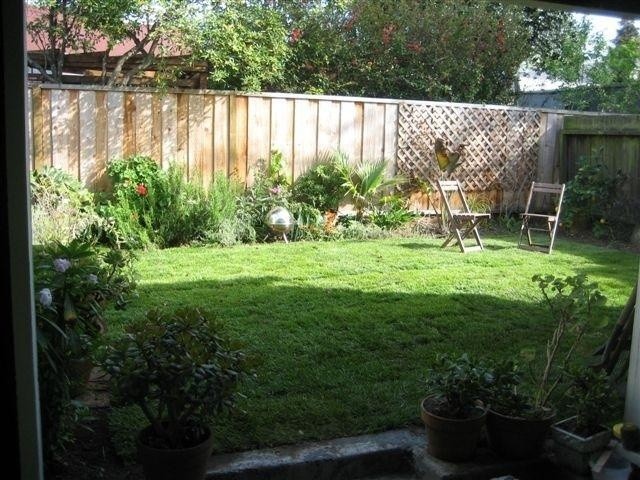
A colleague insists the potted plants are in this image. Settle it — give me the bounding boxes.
[91,309,246,479]
[549,364,611,474]
[422,353,518,462]
[485,272,608,460]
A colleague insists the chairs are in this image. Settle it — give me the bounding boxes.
[518,182,565,254]
[437,180,492,251]
